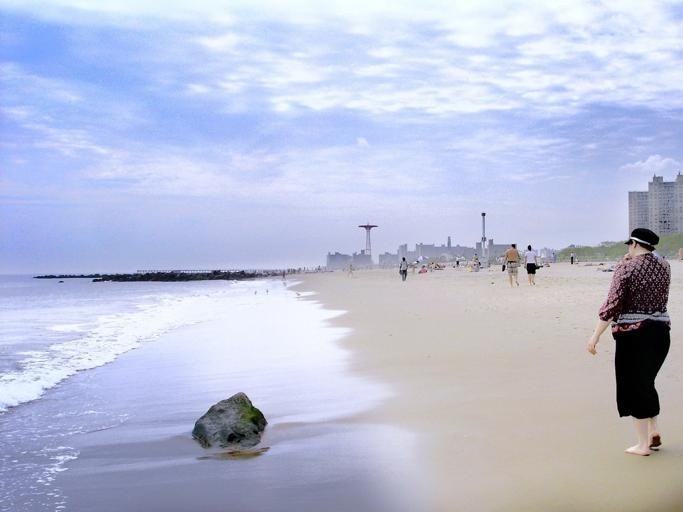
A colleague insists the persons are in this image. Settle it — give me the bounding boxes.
[586,227,671,458]
[502,243,520,288]
[399,256,408,282]
[524,244,537,286]
[415,250,580,274]
[249,263,358,282]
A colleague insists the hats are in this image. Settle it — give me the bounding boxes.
[623,228,660,252]
